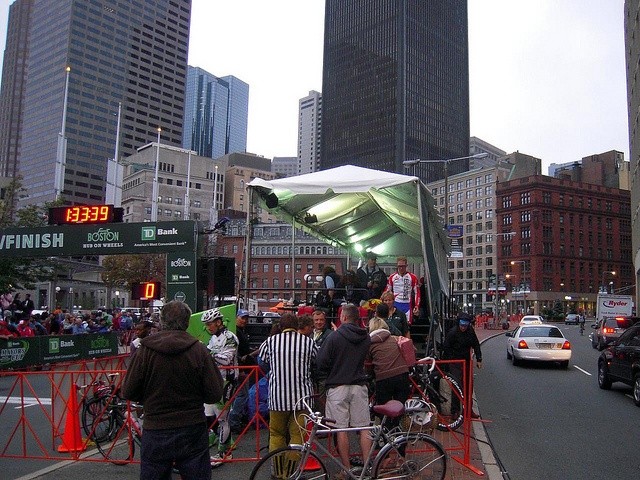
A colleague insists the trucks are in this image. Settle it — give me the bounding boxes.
[211,296,259,324]
[595,294,632,328]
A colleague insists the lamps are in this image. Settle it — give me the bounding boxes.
[305,213,318,224]
[265,192,278,209]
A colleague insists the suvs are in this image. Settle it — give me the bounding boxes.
[597,322,640,407]
[591,316,633,351]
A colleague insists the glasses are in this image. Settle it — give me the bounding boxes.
[398,265,406,267]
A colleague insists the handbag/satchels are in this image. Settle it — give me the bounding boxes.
[316,292,331,307]
[397,336,417,366]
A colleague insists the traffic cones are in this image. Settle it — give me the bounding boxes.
[299,420,321,470]
[56,383,88,452]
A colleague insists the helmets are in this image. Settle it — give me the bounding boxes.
[201,308,224,323]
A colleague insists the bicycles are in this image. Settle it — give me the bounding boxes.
[92,387,180,474]
[249,393,449,480]
[76,372,126,442]
[579,323,584,336]
[357,356,464,431]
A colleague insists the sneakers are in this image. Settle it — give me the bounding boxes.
[471,409,479,418]
[211,450,233,467]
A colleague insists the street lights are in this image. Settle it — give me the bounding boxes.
[472,293,477,315]
[304,273,324,294]
[55,286,61,294]
[68,287,73,314]
[602,271,615,294]
[151,127,163,222]
[506,274,517,312]
[47,64,73,309]
[402,153,489,236]
[115,290,120,308]
[185,149,192,220]
[104,100,123,313]
[511,261,527,316]
[209,165,218,243]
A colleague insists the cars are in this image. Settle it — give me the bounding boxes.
[519,315,547,326]
[261,311,282,323]
[121,307,147,318]
[505,323,572,368]
[565,313,579,325]
[145,307,161,315]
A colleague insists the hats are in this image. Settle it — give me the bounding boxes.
[366,252,377,259]
[238,309,249,317]
[460,320,470,325]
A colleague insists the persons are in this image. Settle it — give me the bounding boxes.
[298,315,314,337]
[124,301,224,480]
[380,290,410,339]
[443,312,482,419]
[0,290,88,371]
[322,266,344,305]
[368,316,417,470]
[256,314,319,480]
[316,305,374,479]
[129,321,155,357]
[578,313,586,330]
[380,257,421,324]
[200,308,240,467]
[334,269,357,305]
[88,309,137,334]
[229,308,259,434]
[377,302,402,336]
[308,310,333,439]
[357,251,388,301]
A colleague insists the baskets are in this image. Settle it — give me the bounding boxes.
[400,408,438,432]
